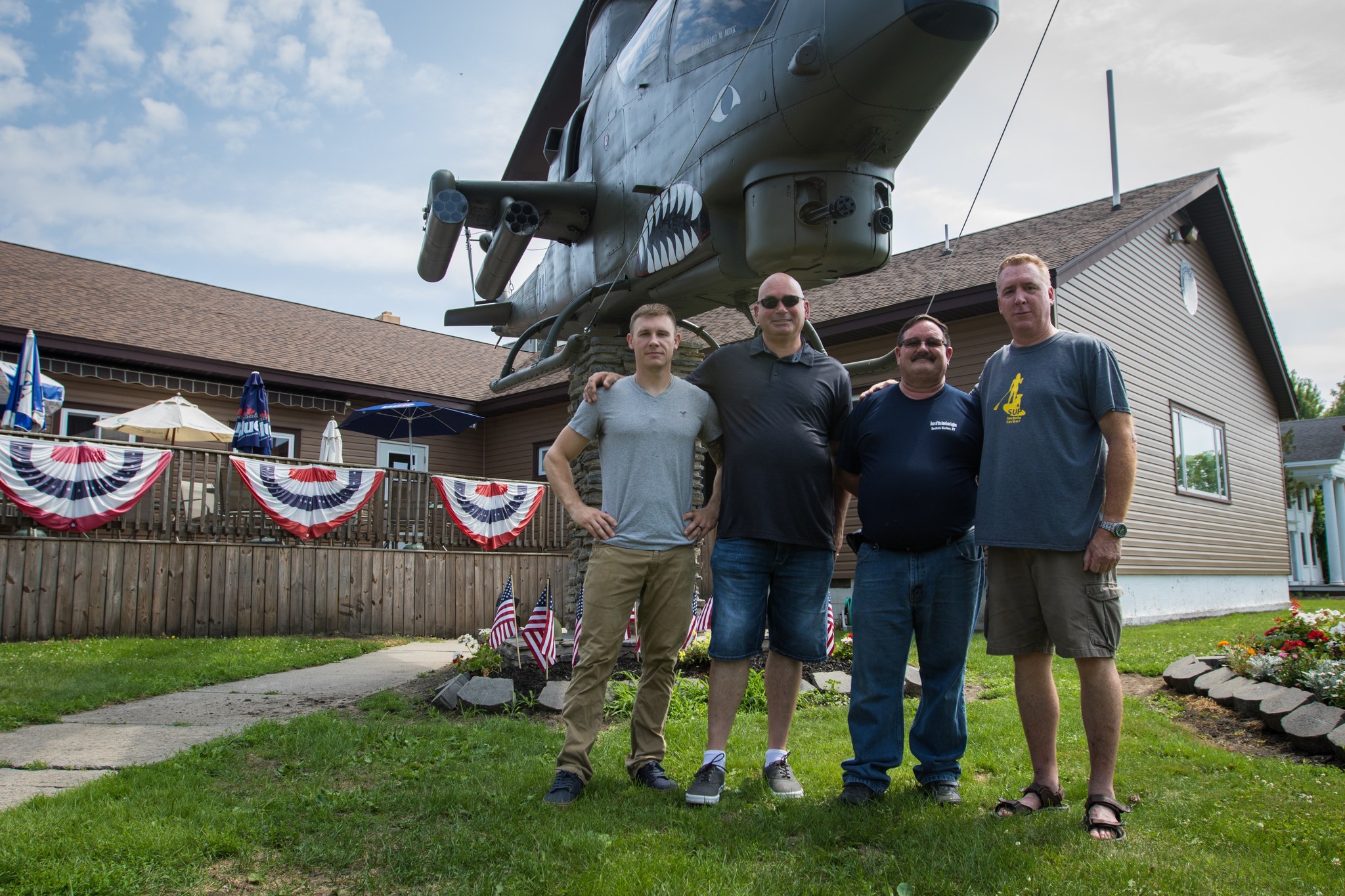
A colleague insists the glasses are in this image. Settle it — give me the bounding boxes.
[901,337,948,349]
[758,295,805,309]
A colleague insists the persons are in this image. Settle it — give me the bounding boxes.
[860,252,1137,843]
[582,273,852,807]
[543,299,724,803]
[836,312,985,810]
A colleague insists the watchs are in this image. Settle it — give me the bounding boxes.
[1097,520,1127,539]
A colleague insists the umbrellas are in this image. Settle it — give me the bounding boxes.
[232,370,272,457]
[1,329,48,432]
[93,392,235,446]
[334,399,485,472]
[319,415,343,465]
[0,359,65,415]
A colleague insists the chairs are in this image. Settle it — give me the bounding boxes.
[343,480,459,546]
[220,459,272,543]
[179,481,226,541]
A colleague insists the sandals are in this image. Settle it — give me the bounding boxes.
[1083,794,1131,844]
[993,782,1068,819]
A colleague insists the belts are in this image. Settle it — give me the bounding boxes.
[866,524,975,553]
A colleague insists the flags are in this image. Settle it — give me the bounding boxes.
[429,474,546,551]
[228,454,386,540]
[489,573,520,655]
[677,581,714,651]
[0,435,173,531]
[571,580,582,668]
[521,578,557,673]
[621,600,642,653]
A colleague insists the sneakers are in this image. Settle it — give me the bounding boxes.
[836,782,875,807]
[762,751,804,800]
[542,768,584,807]
[926,780,962,805]
[632,761,677,792]
[686,753,730,805]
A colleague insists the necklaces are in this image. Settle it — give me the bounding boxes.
[633,371,672,391]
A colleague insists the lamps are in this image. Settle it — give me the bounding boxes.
[1181,224,1199,243]
[1169,229,1184,243]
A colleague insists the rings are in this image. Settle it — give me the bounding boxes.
[837,553,839,556]
[697,525,702,531]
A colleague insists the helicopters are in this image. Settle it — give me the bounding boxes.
[416,1,1006,395]
